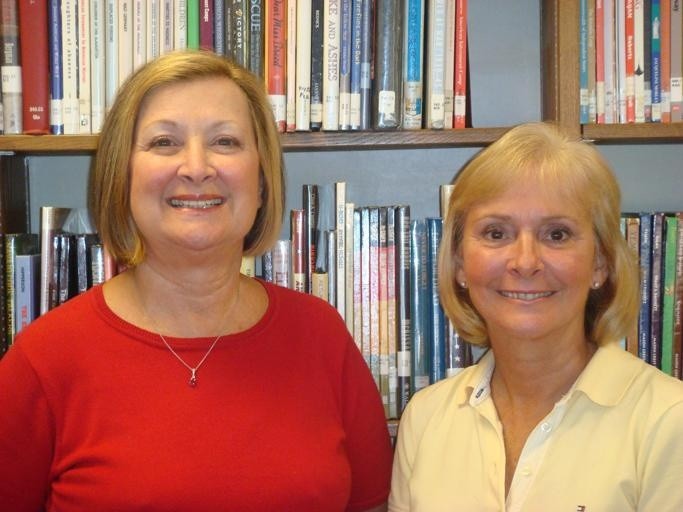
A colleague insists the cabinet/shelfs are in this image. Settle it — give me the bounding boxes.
[0,122,683,443]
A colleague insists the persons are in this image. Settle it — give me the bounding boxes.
[0,49,392,512]
[383,120,683,512]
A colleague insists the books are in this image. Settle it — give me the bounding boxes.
[2,1,472,136]
[4,207,126,350]
[542,1,682,124]
[239,182,488,419]
[614,211,683,382]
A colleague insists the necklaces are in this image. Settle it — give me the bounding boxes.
[144,307,232,384]
